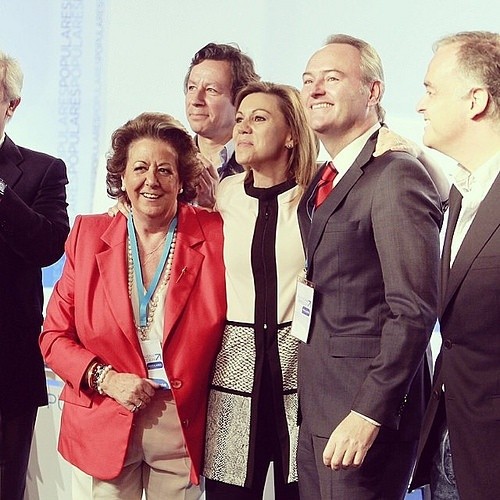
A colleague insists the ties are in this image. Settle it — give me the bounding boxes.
[314,161,338,208]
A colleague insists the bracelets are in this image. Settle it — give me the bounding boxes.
[87,362,111,394]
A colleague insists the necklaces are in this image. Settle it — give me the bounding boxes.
[128,227,177,341]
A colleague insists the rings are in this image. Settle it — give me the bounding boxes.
[131,402,142,413]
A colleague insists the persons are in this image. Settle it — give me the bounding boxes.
[183,42,262,181]
[373,32,500,500]
[295,34,445,500]
[37,113,227,500]
[109,82,321,500]
[0,50,70,500]
